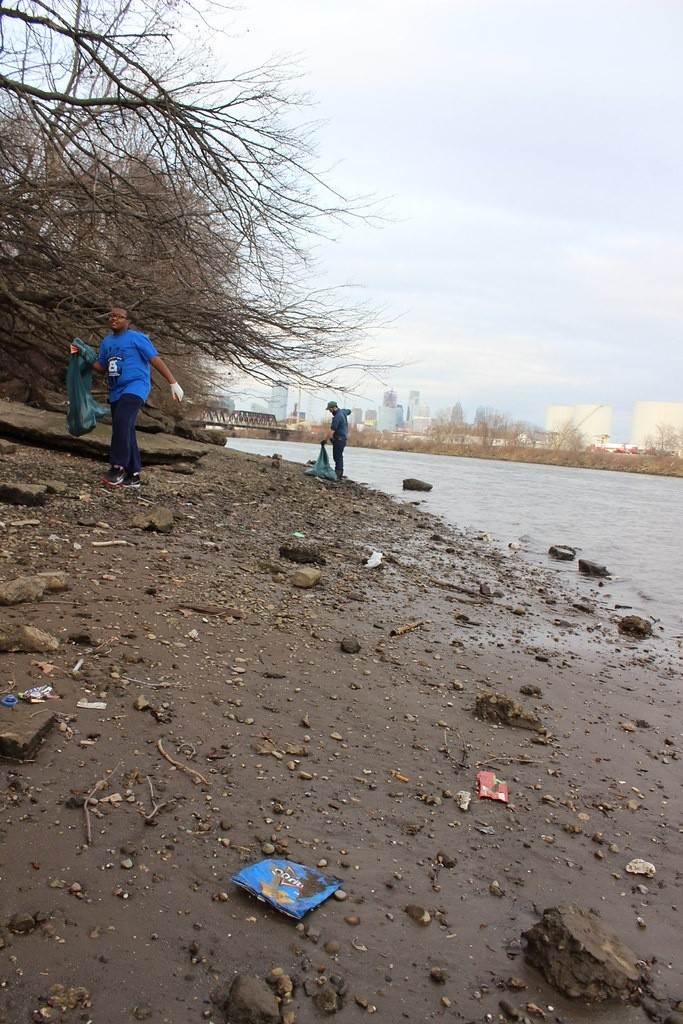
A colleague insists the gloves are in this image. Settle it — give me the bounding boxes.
[169,381,184,403]
[70,344,78,354]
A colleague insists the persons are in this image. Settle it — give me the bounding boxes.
[319,400,351,483]
[67,304,186,491]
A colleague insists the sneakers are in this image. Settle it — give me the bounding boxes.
[102,464,126,485]
[118,472,141,488]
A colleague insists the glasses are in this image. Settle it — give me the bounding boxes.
[107,313,128,321]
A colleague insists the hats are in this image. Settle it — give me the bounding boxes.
[325,401,337,410]
